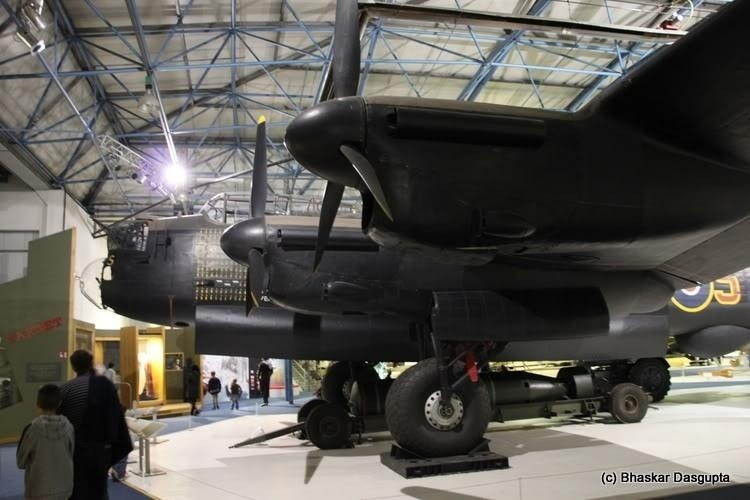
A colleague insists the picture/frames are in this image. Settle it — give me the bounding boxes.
[165,352,184,371]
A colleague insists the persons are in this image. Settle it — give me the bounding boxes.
[229,379,241,410]
[102,362,116,393]
[14,383,78,500]
[186,364,201,416]
[207,371,221,410]
[53,348,135,500]
[257,357,273,407]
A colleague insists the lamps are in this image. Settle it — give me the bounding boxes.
[15,0,48,57]
[95,135,176,205]
[137,76,161,114]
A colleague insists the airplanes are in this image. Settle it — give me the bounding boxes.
[93,0,749,455]
